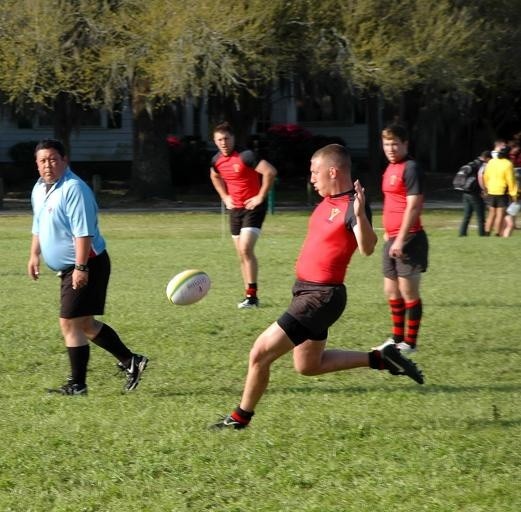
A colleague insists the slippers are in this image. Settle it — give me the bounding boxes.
[452,159,482,192]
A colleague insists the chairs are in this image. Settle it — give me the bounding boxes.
[384,344,424,384]
[210,417,244,430]
[113,353,149,394]
[372,338,397,350]
[395,341,416,351]
[236,295,258,309]
[47,377,88,397]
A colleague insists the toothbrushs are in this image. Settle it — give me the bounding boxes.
[166,269,210,305]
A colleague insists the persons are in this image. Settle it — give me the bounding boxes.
[458,138,521,238]
[380,127,429,351]
[210,121,279,309]
[208,144,425,429]
[28,137,148,395]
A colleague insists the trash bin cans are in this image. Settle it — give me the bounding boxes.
[74,265,90,271]
